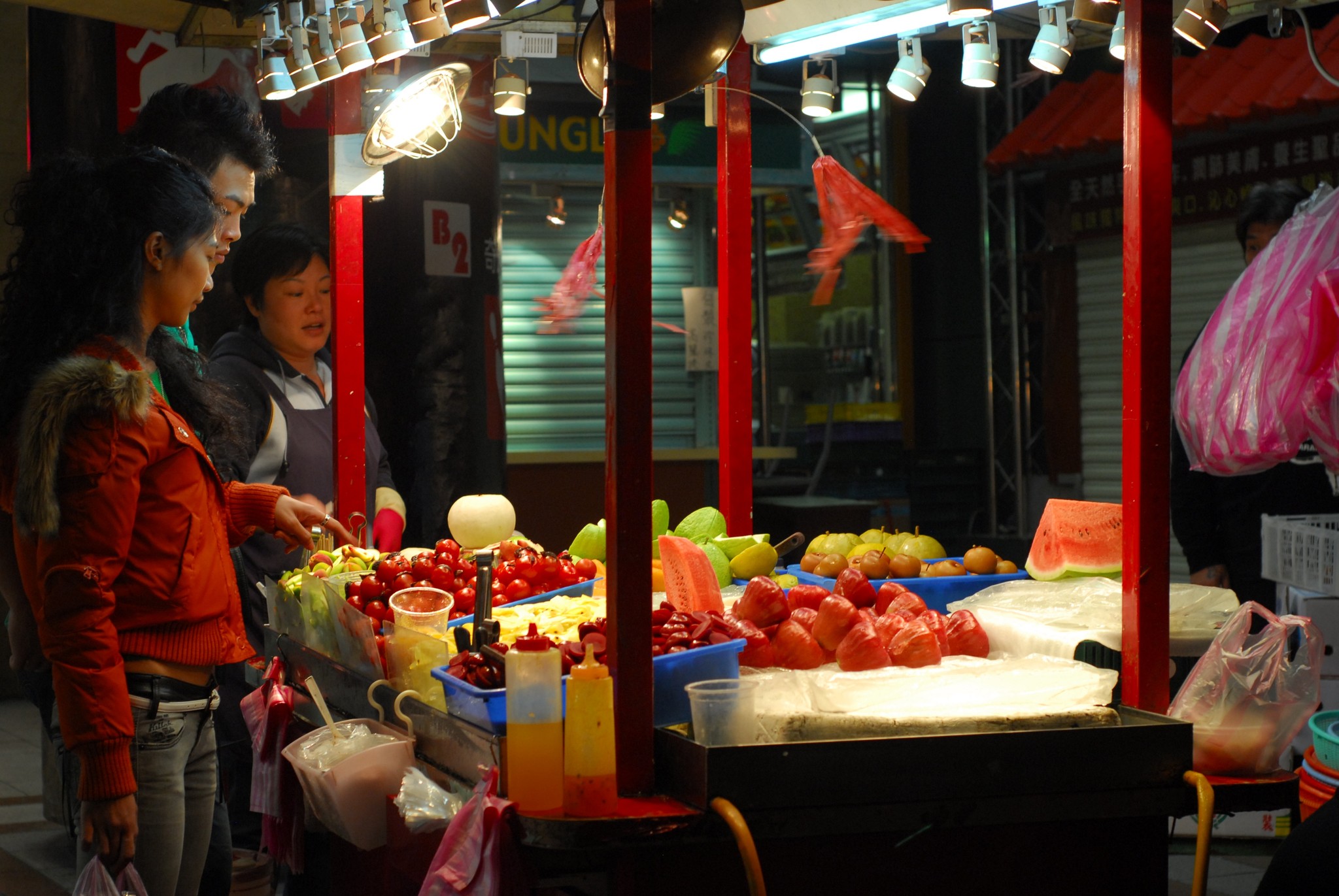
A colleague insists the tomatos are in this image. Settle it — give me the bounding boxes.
[345,539,596,678]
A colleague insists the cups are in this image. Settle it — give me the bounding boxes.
[683,678,761,747]
[328,570,377,600]
[388,587,455,692]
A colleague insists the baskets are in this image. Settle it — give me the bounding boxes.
[1261,513,1339,596]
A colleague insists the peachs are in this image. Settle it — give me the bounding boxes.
[276,544,383,659]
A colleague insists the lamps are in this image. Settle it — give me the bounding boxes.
[250,0,1228,230]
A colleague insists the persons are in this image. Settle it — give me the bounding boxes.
[0,82,359,896]
[203,227,409,875]
[1169,189,1338,636]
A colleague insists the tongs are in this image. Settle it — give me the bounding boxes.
[299,526,322,569]
[344,511,367,547]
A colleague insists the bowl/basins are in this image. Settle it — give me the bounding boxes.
[1178,724,1274,776]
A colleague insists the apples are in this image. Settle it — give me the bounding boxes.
[801,547,1018,579]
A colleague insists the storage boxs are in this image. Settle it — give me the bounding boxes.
[1260,513,1339,597]
[328,557,1027,727]
[1275,583,1339,755]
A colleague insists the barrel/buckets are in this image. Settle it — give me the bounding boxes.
[229,847,274,896]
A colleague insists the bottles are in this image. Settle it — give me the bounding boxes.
[563,644,618,818]
[505,621,563,811]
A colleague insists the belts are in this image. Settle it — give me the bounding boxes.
[128,688,220,712]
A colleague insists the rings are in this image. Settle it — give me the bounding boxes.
[311,526,321,542]
[319,513,330,527]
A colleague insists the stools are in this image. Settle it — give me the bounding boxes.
[1185,769,1301,896]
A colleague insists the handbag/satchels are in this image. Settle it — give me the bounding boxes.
[1166,601,1324,777]
[1171,184,1338,476]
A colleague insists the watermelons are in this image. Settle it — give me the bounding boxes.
[1025,498,1123,580]
[657,536,723,616]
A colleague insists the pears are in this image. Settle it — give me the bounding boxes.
[569,518,606,566]
[805,526,947,562]
[652,499,798,592]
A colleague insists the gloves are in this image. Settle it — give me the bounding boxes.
[371,508,404,553]
